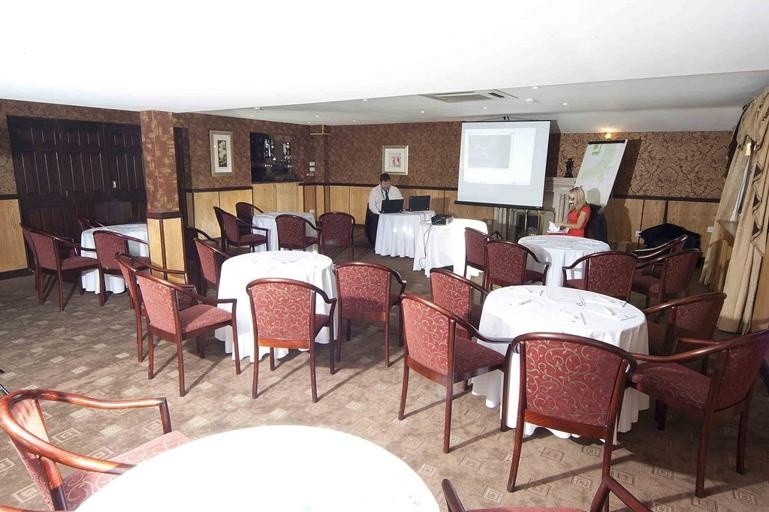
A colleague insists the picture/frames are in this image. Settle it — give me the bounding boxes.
[210,129,235,178]
[381,144,409,176]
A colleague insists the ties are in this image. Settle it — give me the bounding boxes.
[386,191,389,201]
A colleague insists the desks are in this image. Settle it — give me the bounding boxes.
[414,219,489,279]
[469,285,650,445]
[74,426,442,512]
[214,249,339,360]
[374,211,435,260]
[518,235,610,286]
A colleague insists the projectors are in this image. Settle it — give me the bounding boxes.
[431,214,454,225]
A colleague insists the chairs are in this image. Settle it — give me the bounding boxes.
[444,475,651,512]
[562,234,703,306]
[182,228,233,296]
[134,270,241,396]
[19,217,149,307]
[115,252,206,362]
[246,278,337,402]
[213,202,356,258]
[0,388,190,512]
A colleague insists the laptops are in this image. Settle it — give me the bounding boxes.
[379,199,404,213]
[405,195,430,211]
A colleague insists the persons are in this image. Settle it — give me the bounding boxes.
[368,173,402,215]
[554,186,590,237]
